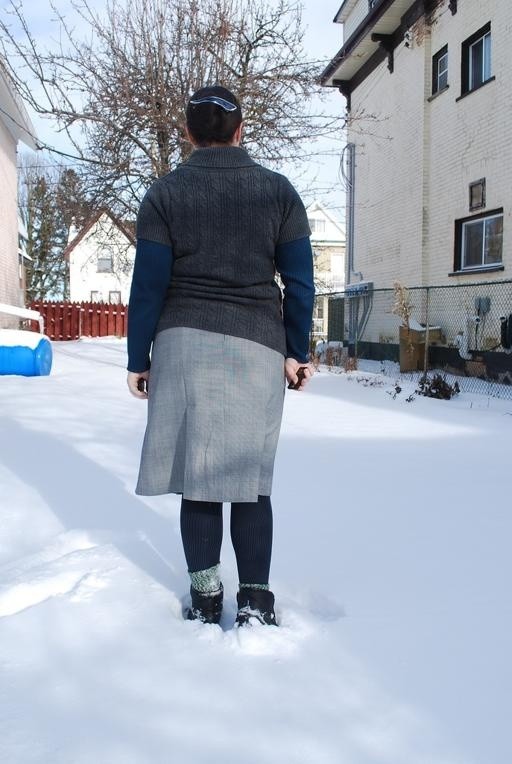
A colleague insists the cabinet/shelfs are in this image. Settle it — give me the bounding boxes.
[398,322,441,372]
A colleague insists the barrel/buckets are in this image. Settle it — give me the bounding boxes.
[329,341,343,349]
[0,335,53,376]
[337,347,348,366]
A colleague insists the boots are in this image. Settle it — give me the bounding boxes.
[184,584,223,625]
[234,589,278,629]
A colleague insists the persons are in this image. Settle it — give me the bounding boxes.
[126,86,316,628]
